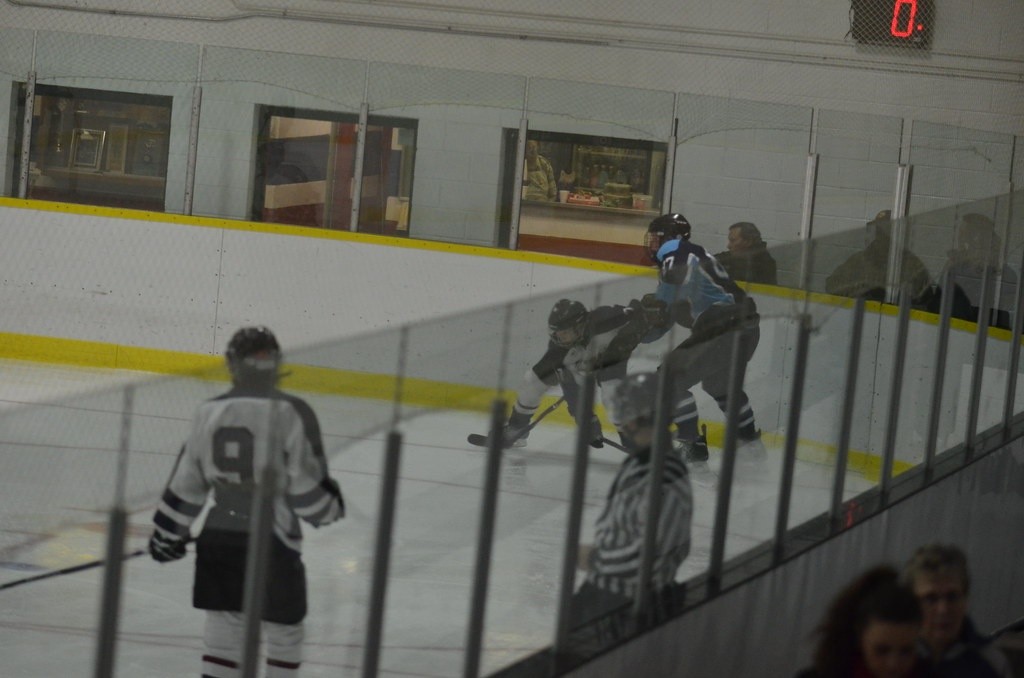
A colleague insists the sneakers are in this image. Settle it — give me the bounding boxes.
[674,423,709,472]
[489,425,528,447]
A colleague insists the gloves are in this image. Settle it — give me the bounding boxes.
[149,532,184,561]
[588,415,604,450]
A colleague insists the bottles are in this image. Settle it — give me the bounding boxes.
[583,164,625,189]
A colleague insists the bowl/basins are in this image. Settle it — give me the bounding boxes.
[602,192,632,207]
[604,183,632,193]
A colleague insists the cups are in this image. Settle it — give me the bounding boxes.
[559,190,570,203]
[632,194,652,210]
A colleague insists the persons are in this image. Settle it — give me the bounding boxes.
[827,211,928,307]
[919,214,1017,333]
[570,371,695,620]
[523,141,556,203]
[792,563,938,678]
[489,214,767,473]
[712,222,778,284]
[149,328,345,678]
[904,541,997,678]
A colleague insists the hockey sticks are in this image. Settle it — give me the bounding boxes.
[0,536,199,593]
[467,394,566,450]
[604,437,631,455]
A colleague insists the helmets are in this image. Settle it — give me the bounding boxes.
[612,373,675,424]
[547,300,591,352]
[644,213,690,276]
[225,327,280,385]
